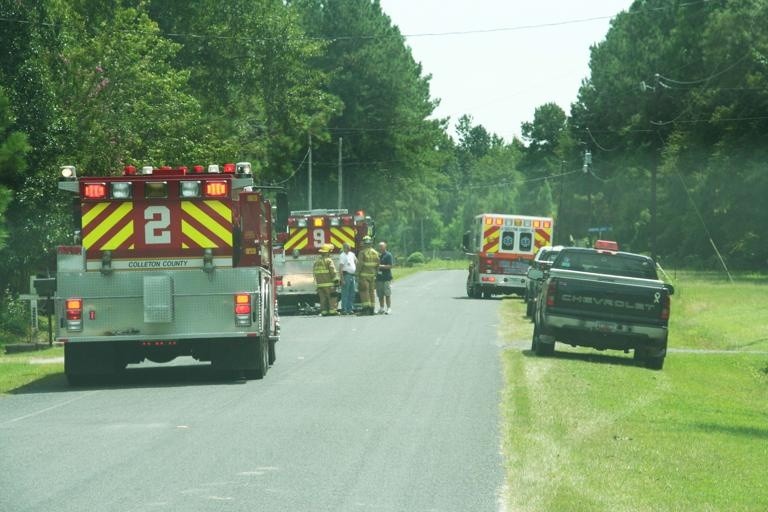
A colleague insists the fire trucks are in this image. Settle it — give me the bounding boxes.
[463,213,554,298]
[273,208,375,298]
[56,161,280,380]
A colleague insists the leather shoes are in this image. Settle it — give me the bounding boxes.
[322,307,374,316]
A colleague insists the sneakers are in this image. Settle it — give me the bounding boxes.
[376,309,392,315]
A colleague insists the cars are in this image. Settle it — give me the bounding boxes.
[526,241,672,368]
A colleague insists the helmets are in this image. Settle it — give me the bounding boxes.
[318,243,335,253]
[362,236,372,244]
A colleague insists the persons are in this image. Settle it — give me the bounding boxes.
[313,236,393,316]
